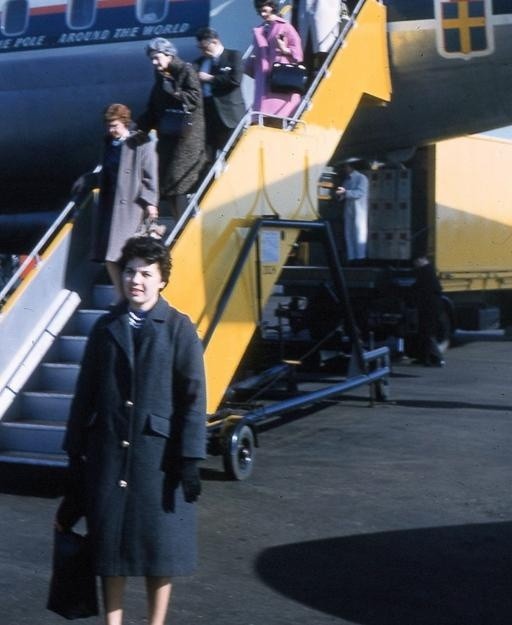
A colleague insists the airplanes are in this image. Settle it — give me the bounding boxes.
[2,0,512,471]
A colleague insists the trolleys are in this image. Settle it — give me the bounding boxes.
[202,217,392,480]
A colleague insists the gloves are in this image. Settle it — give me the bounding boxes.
[169,460,202,503]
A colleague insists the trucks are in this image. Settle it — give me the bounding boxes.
[275,134,512,363]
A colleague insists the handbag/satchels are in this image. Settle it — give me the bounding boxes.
[269,54,310,94]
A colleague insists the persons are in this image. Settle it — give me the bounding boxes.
[295,0,347,80]
[241,0,304,126]
[189,26,247,154]
[63,235,207,625]
[334,164,373,264]
[70,103,160,307]
[133,38,204,221]
